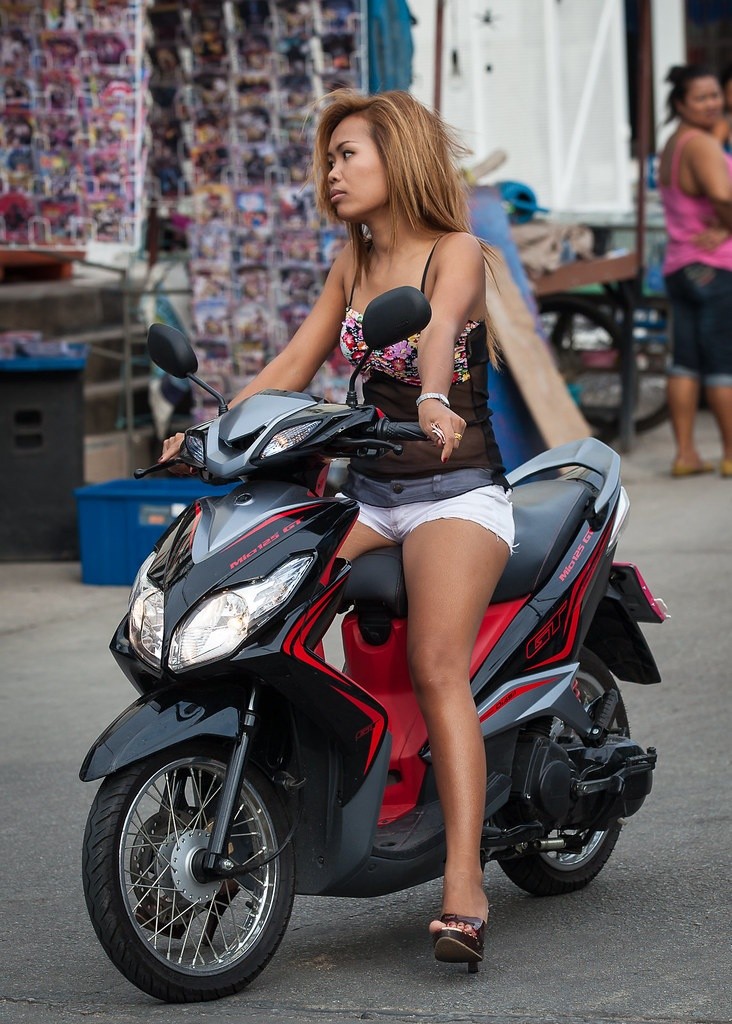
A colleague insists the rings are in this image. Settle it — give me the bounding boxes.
[454,432,463,440]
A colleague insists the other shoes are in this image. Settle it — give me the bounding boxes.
[720,459,732,477]
[673,461,715,476]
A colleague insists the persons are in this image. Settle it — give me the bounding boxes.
[160,90,521,976]
[657,62,732,477]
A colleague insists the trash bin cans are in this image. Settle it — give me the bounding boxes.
[0,355,87,561]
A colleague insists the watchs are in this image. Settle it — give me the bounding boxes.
[416,393,451,408]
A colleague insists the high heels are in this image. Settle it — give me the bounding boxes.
[135,885,241,946]
[434,913,485,973]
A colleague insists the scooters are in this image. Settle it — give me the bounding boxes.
[80,287,672,1005]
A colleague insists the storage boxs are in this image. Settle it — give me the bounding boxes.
[74,479,233,586]
